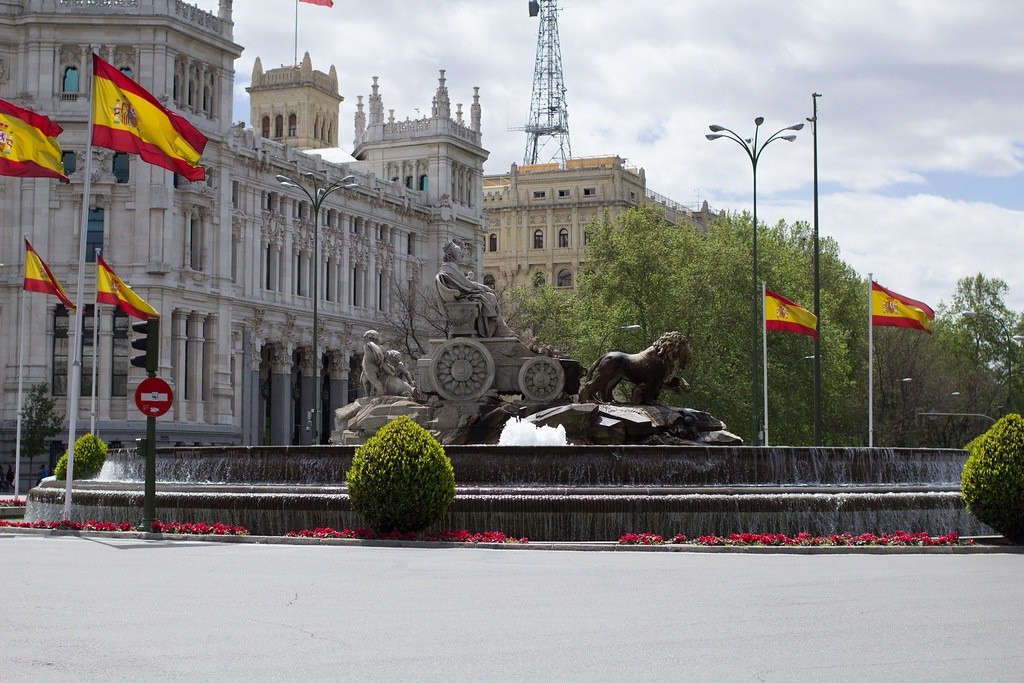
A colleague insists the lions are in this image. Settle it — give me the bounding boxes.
[585,331,692,405]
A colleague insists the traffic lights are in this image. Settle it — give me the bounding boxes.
[131,318,160,372]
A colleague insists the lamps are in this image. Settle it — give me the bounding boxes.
[321,389,329,401]
[292,388,300,400]
[261,385,270,400]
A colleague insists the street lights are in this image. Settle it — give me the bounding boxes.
[276,171,359,446]
[704,116,807,445]
[787,354,823,445]
[962,311,1011,416]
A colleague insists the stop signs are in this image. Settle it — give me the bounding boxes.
[135,378,173,418]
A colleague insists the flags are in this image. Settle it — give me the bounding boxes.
[90,52,209,182]
[1,100,70,182]
[765,288,819,341]
[22,239,76,309]
[299,1,335,8]
[96,255,161,321]
[872,280,935,335]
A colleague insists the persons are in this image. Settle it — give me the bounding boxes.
[359,330,416,397]
[439,241,516,338]
